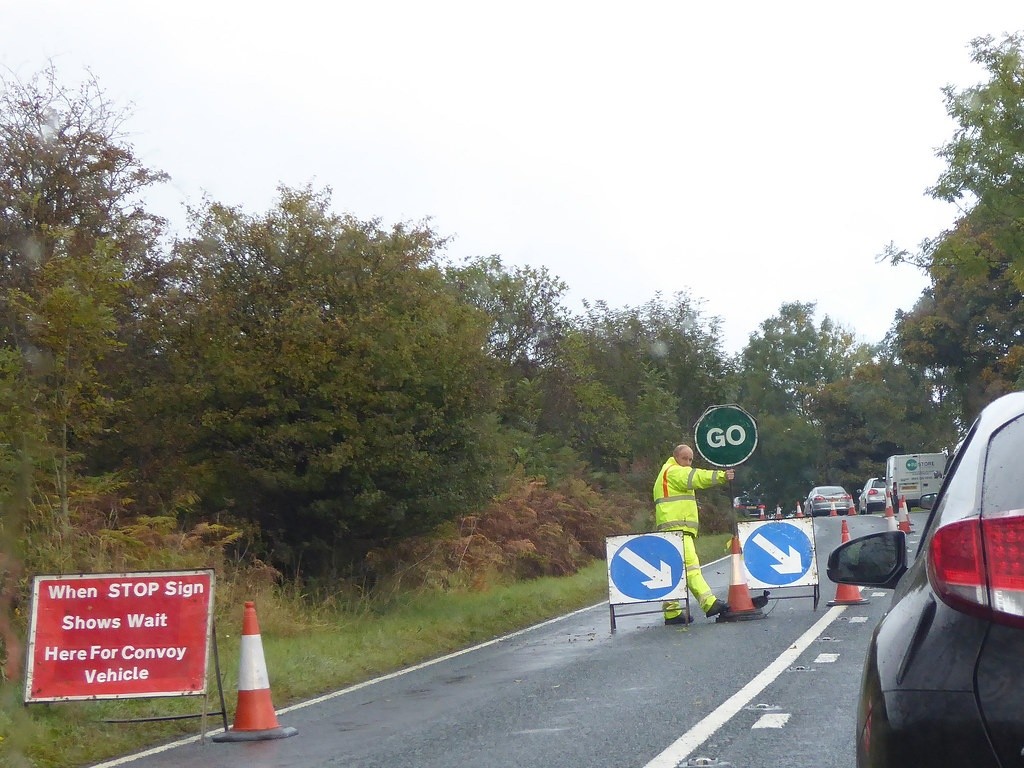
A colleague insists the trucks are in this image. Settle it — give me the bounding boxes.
[885,451,947,510]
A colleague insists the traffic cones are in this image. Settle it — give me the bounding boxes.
[716,535,766,619]
[752,488,925,609]
[214,595,296,742]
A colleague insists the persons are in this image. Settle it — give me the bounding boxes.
[652,443,736,624]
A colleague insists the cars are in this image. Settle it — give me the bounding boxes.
[858,474,888,517]
[803,486,854,516]
[853,384,1024,768]
[734,494,765,519]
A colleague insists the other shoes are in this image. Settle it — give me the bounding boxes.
[705,599,731,617]
[665,611,695,625]
